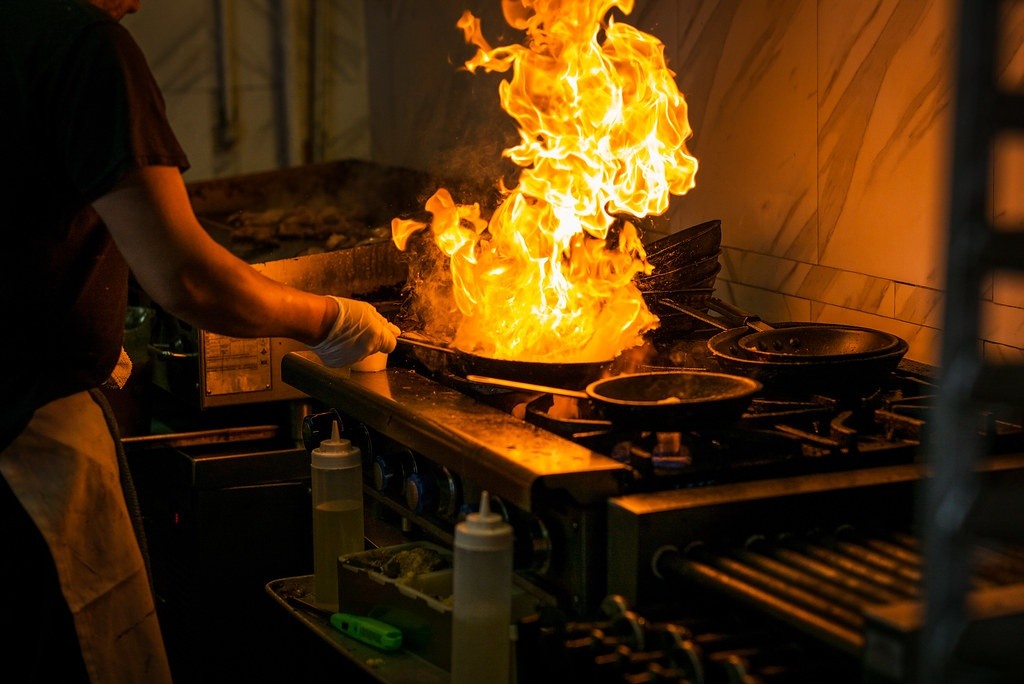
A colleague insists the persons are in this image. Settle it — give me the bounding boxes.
[0,0,403,684]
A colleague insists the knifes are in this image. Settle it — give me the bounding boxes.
[283,594,403,650]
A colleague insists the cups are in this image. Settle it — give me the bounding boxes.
[122,306,155,378]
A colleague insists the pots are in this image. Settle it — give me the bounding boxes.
[658,295,910,384]
[701,297,898,359]
[586,369,762,431]
